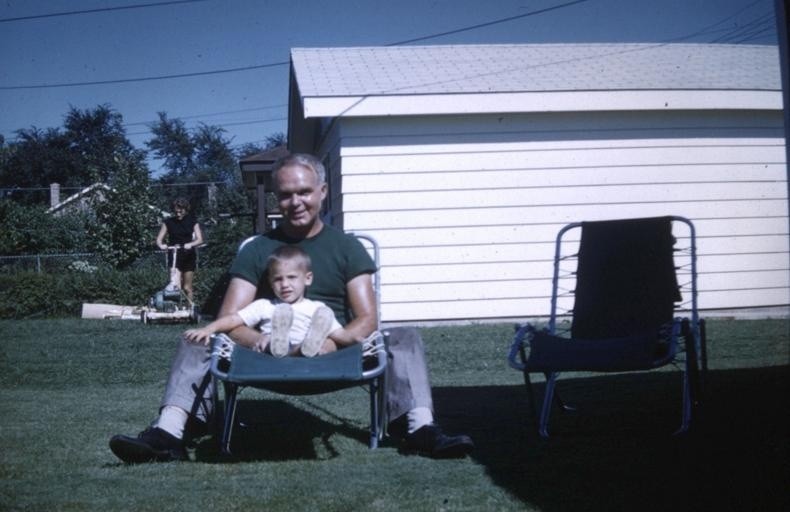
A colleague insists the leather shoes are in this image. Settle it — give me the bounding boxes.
[395,422,472,465]
[108,424,191,466]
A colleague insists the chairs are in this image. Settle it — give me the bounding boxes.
[213,233,386,453]
[510,216,707,456]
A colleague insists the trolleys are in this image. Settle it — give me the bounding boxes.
[140,243,202,325]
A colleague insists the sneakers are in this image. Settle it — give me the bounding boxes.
[269,302,295,361]
[299,303,336,358]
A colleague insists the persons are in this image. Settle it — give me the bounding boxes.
[107,151,476,467]
[183,245,367,358]
[155,197,205,301]
